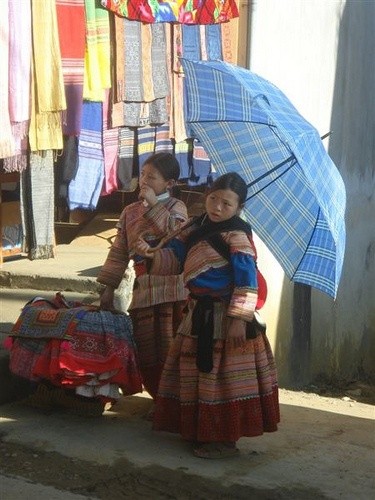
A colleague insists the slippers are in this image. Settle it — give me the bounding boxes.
[147,403,154,420]
[181,433,198,443]
[193,440,240,459]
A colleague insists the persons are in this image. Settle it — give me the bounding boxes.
[134,172,282,462]
[97,152,191,423]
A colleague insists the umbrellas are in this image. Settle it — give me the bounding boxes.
[177,57,349,304]
